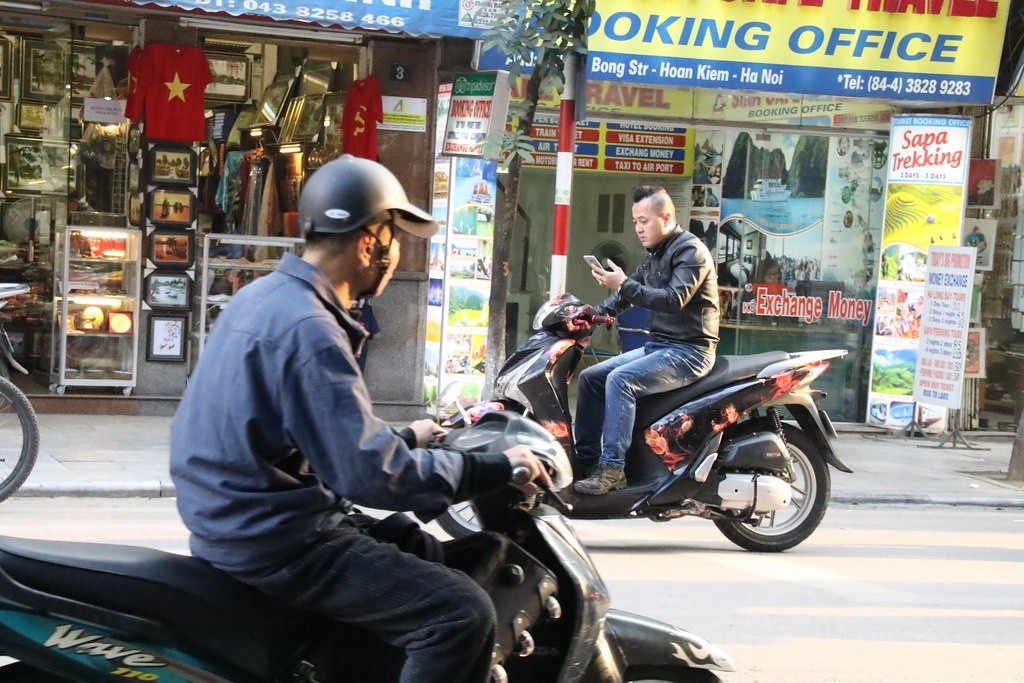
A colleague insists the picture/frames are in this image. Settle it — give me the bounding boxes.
[204,50,252,104]
[58,314,74,332]
[4,133,82,197]
[143,269,193,312]
[18,35,134,110]
[249,57,346,149]
[146,185,196,230]
[16,101,56,136]
[148,142,197,188]
[148,227,195,270]
[146,313,188,363]
[0,35,15,104]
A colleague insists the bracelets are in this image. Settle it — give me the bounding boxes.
[616,276,629,293]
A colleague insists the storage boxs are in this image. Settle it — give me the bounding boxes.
[109,312,133,334]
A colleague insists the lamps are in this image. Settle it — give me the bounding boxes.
[279,145,302,154]
[179,17,364,47]
[250,131,262,136]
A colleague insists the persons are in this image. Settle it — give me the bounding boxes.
[169,153,555,682]
[571,184,721,494]
[683,121,925,336]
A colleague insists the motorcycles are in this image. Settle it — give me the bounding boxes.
[1,362,739,683]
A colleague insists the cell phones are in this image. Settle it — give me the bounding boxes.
[584,255,604,274]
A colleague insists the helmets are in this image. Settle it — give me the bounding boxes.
[299,153,435,233]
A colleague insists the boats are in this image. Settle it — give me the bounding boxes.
[749,176,791,203]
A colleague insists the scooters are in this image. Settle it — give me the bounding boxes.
[1,282,41,505]
[433,289,855,553]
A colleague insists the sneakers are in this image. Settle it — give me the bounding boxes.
[574,453,600,479]
[574,463,628,495]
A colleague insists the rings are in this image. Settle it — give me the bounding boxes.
[598,280,602,285]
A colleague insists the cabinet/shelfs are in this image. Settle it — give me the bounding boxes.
[198,231,307,356]
[47,224,142,396]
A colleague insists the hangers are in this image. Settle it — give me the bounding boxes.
[356,72,368,86]
